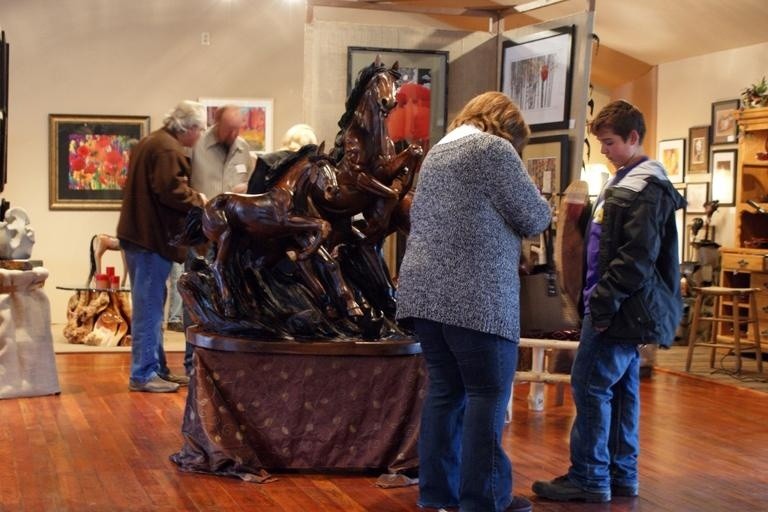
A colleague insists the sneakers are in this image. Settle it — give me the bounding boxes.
[129,374,190,392]
[504,474,638,512]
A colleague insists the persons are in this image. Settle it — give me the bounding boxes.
[181,106,255,378]
[116,101,209,392]
[395,90,551,511]
[532,102,684,502]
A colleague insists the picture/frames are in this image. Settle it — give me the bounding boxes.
[197,97,275,157]
[346,45,450,150]
[519,134,571,236]
[47,114,152,212]
[658,125,712,264]
[500,23,575,130]
[712,148,736,206]
[713,100,738,145]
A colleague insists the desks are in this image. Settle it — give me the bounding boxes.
[184,326,423,488]
[56,284,132,346]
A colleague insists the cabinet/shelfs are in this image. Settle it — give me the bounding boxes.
[718,107,768,354]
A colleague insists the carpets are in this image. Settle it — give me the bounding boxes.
[656,346,768,395]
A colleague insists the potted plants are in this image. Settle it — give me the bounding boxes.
[741,73,768,108]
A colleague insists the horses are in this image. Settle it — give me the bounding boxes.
[263,54,424,317]
[167,140,342,319]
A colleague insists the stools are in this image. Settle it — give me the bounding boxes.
[504,336,580,425]
[683,285,762,374]
